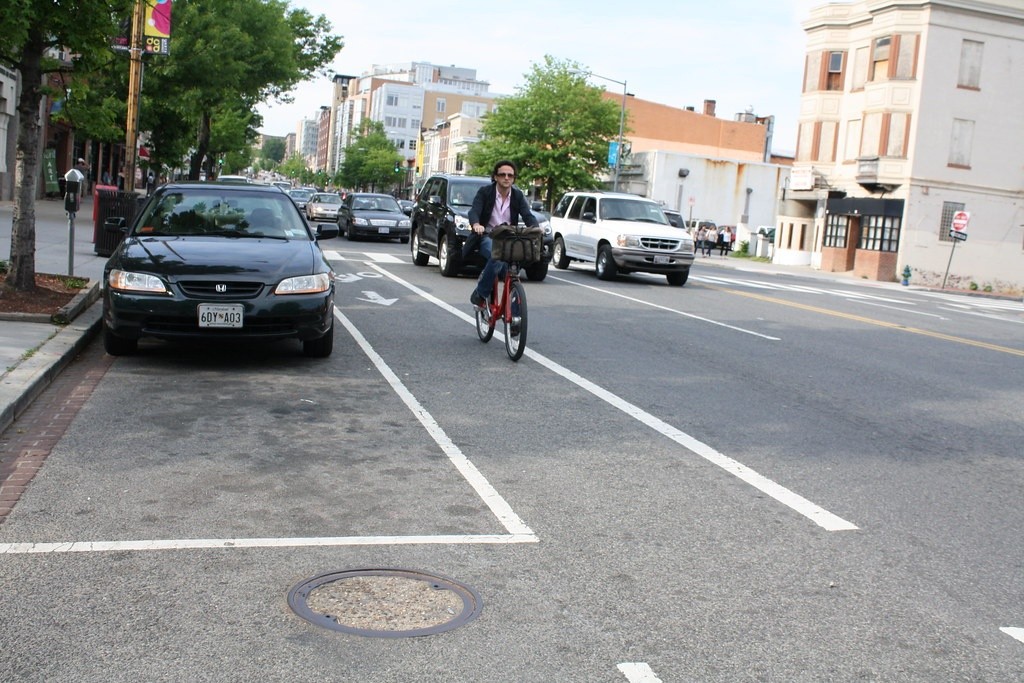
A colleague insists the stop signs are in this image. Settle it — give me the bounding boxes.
[950,210,970,233]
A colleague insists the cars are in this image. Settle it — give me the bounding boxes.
[685,219,776,257]
[651,209,693,241]
[100,184,341,361]
[215,172,415,244]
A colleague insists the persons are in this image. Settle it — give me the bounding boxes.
[694,224,718,259]
[74,157,90,201]
[145,172,155,197]
[103,166,124,190]
[468,160,543,337]
[719,226,732,256]
[456,191,466,203]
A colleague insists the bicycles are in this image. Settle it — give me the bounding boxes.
[473,225,548,364]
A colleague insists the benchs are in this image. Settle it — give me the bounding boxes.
[200,213,245,229]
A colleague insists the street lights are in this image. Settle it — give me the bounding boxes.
[566,67,628,192]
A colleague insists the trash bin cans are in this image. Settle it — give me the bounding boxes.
[94,188,140,258]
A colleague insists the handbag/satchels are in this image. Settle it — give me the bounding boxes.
[489,222,542,259]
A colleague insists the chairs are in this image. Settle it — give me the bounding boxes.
[251,208,276,226]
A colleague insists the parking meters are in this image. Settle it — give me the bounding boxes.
[63,168,85,276]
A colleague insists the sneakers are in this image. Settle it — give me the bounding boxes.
[470,290,487,309]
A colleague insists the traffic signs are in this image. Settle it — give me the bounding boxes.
[948,230,967,241]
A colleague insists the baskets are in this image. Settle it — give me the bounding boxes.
[492,221,543,262]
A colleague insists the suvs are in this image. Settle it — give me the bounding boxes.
[412,171,555,282]
[549,189,697,286]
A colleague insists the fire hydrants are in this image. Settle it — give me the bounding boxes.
[901,264,911,285]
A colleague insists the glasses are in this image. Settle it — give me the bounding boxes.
[496,173,514,177]
